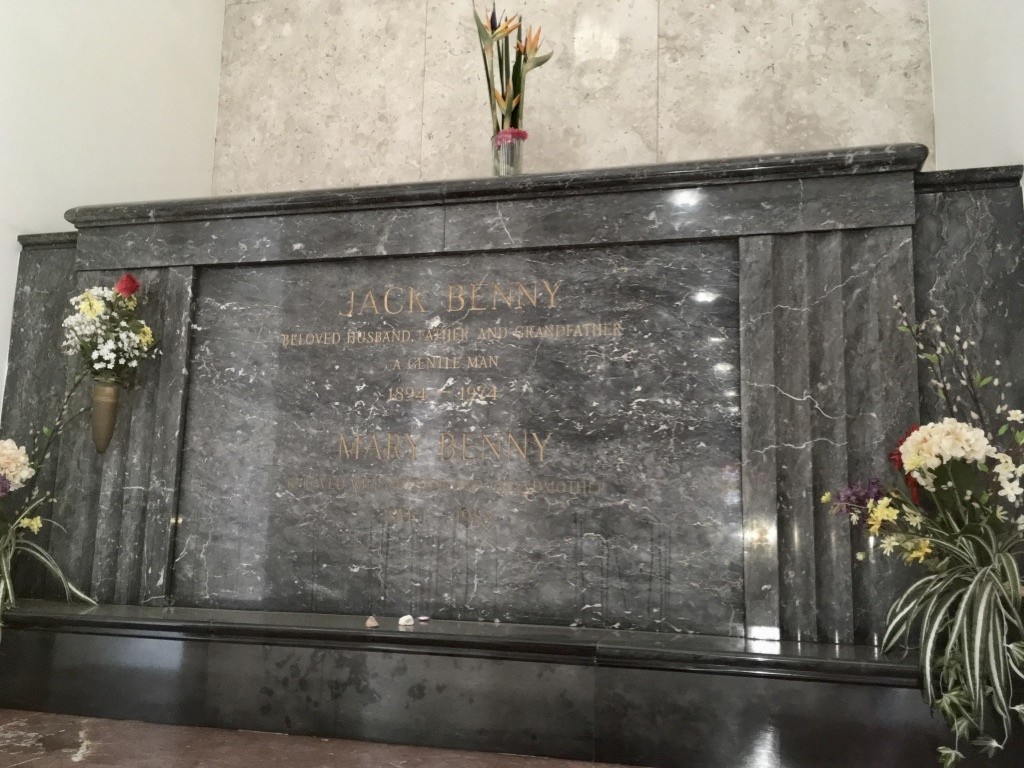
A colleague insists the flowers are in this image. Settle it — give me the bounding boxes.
[472,0,554,149]
[819,294,1024,768]
[0,371,97,613]
[61,274,164,395]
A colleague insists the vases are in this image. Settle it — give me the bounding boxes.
[489,134,522,177]
[91,377,120,454]
